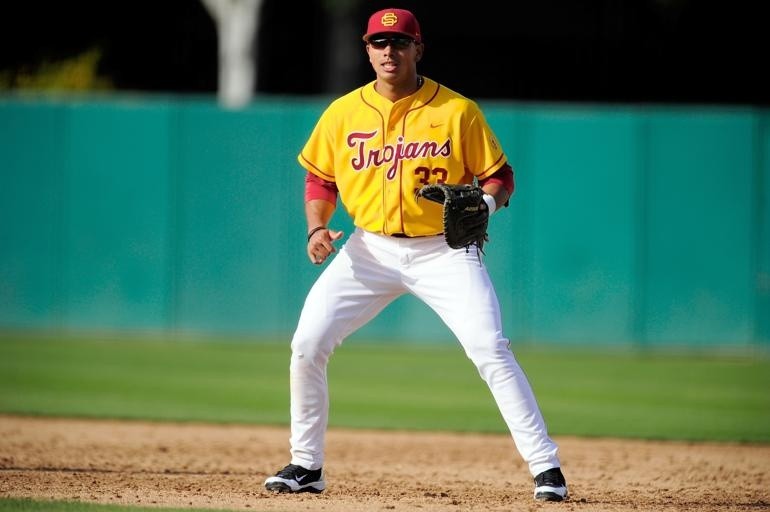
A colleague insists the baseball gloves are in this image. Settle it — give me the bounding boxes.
[419,182,488,251]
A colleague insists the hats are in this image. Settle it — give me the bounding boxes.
[363,8,421,43]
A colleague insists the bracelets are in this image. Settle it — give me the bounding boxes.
[306,226,327,241]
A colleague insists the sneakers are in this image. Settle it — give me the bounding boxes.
[533,467,567,501]
[264,461,325,493]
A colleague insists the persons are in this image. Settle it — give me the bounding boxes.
[263,7,570,502]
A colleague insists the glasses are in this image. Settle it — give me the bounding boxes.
[369,36,412,49]
